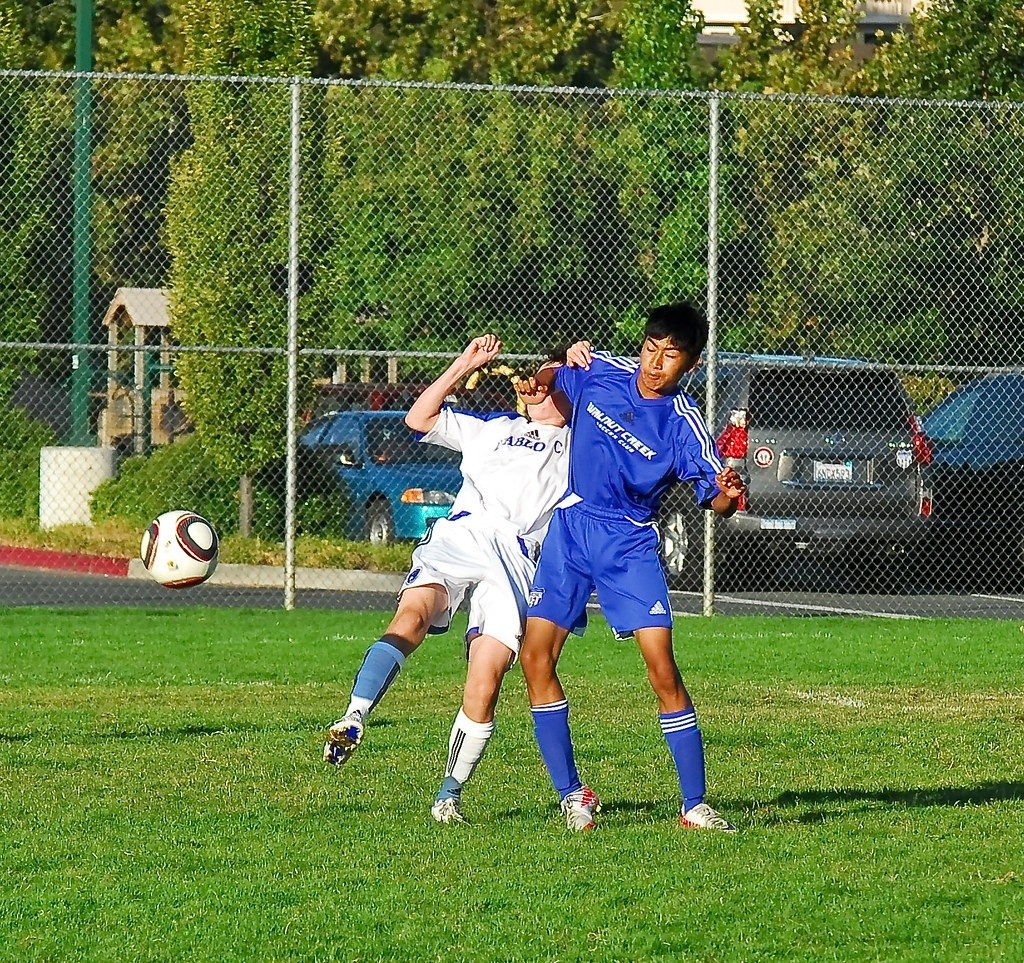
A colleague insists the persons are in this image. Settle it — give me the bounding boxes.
[321,333,592,826]
[513,300,748,837]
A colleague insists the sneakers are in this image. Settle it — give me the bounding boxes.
[431,797,469,826]
[681,803,739,833]
[560,786,602,832]
[324,712,366,770]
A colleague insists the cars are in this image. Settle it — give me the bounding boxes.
[297,411,464,547]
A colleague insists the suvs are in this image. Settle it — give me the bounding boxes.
[655,352,934,591]
[922,372,1023,590]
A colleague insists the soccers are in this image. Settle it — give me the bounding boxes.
[140,509,220,590]
